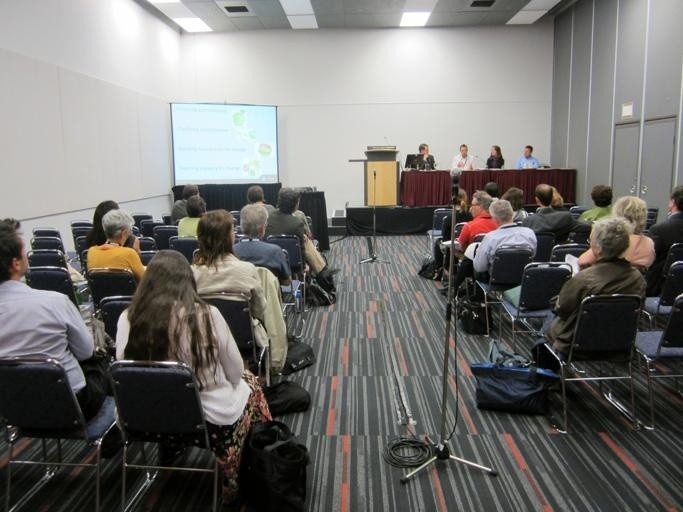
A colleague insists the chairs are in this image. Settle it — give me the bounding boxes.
[266,234,310,313]
[27,266,81,313]
[138,237,158,251]
[549,243,591,262]
[641,260,683,331]
[30,236,84,272]
[74,236,89,275]
[140,219,162,237]
[70,219,94,227]
[498,261,574,354]
[534,231,559,263]
[0,352,116,512]
[132,225,140,236]
[536,293,643,434]
[571,213,581,221]
[647,211,657,223]
[99,295,134,341]
[168,234,201,265]
[440,221,467,283]
[82,249,90,275]
[85,267,138,315]
[570,205,590,214]
[72,226,94,260]
[426,208,453,260]
[456,233,487,301]
[648,206,659,218]
[106,359,219,512]
[27,249,88,295]
[473,243,534,337]
[132,214,153,228]
[645,219,654,231]
[649,242,683,296]
[608,292,683,431]
[230,210,240,227]
[140,250,159,266]
[32,225,78,262]
[199,292,272,387]
[152,225,178,250]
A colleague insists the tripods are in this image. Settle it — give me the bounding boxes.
[399,204,499,486]
[359,170,390,265]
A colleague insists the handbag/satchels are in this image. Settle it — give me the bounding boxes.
[240,418,312,511]
[470,352,561,418]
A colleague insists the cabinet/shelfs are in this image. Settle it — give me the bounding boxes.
[362,144,400,207]
[458,167,577,207]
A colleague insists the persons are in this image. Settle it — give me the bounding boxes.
[501,188,528,222]
[454,199,537,283]
[240,186,276,236]
[116,250,274,498]
[190,211,269,351]
[578,195,655,267]
[410,143,435,170]
[178,196,207,239]
[171,184,199,226]
[648,185,683,294]
[0,218,114,426]
[521,184,575,241]
[458,191,498,250]
[82,201,140,254]
[516,146,540,169]
[578,185,614,222]
[486,182,498,200]
[87,210,145,283]
[545,215,647,358]
[264,188,306,268]
[452,144,477,170]
[487,146,505,168]
[232,203,292,286]
[433,188,467,277]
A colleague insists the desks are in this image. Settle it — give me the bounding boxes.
[399,168,453,207]
[297,190,329,250]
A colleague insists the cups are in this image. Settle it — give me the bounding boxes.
[425,164,430,171]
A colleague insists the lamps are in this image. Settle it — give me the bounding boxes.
[161,214,173,226]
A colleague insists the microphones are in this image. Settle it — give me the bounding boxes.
[450,167,461,205]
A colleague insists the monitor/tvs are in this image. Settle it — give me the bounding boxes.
[404,154,424,171]
